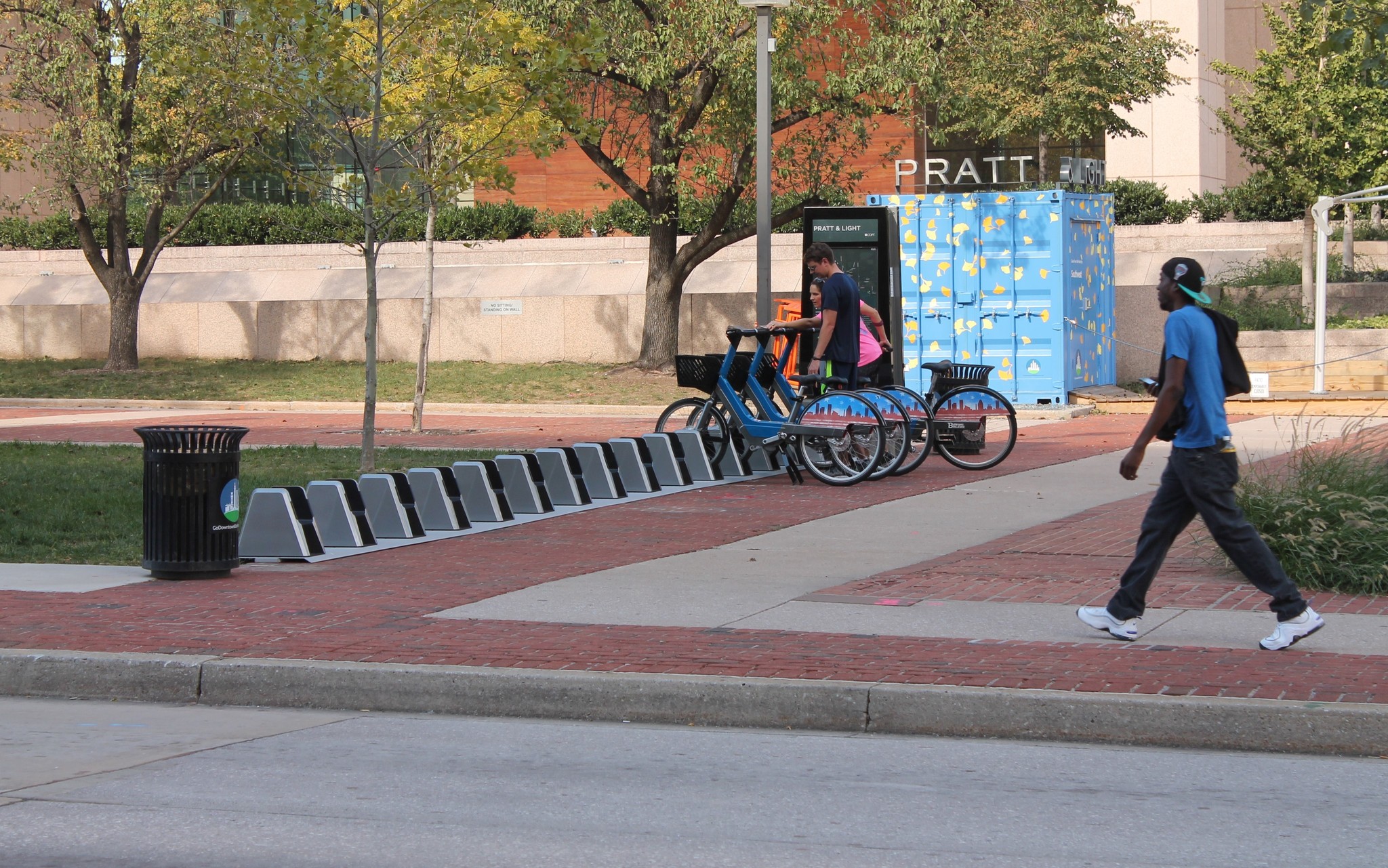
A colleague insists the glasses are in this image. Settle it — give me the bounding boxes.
[807,265,817,271]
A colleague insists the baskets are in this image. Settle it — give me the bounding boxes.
[705,353,751,393]
[675,355,722,395]
[735,351,779,390]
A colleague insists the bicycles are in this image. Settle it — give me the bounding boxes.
[874,343,1019,471]
[655,327,934,487]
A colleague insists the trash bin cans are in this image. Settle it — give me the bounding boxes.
[931,363,995,455]
[133,424,250,579]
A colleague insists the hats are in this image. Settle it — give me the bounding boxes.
[1162,257,1213,303]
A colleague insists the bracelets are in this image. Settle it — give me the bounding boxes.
[813,356,821,361]
[872,318,884,328]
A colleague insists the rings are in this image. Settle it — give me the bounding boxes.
[772,323,774,325]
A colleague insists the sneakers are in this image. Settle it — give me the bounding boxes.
[878,452,895,467]
[1076,606,1142,642]
[854,459,871,472]
[1260,605,1325,651]
[824,467,848,479]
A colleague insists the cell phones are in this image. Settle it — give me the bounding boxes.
[1137,375,1160,389]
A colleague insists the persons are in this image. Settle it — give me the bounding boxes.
[1075,257,1326,649]
[760,277,898,469]
[806,242,877,478]
